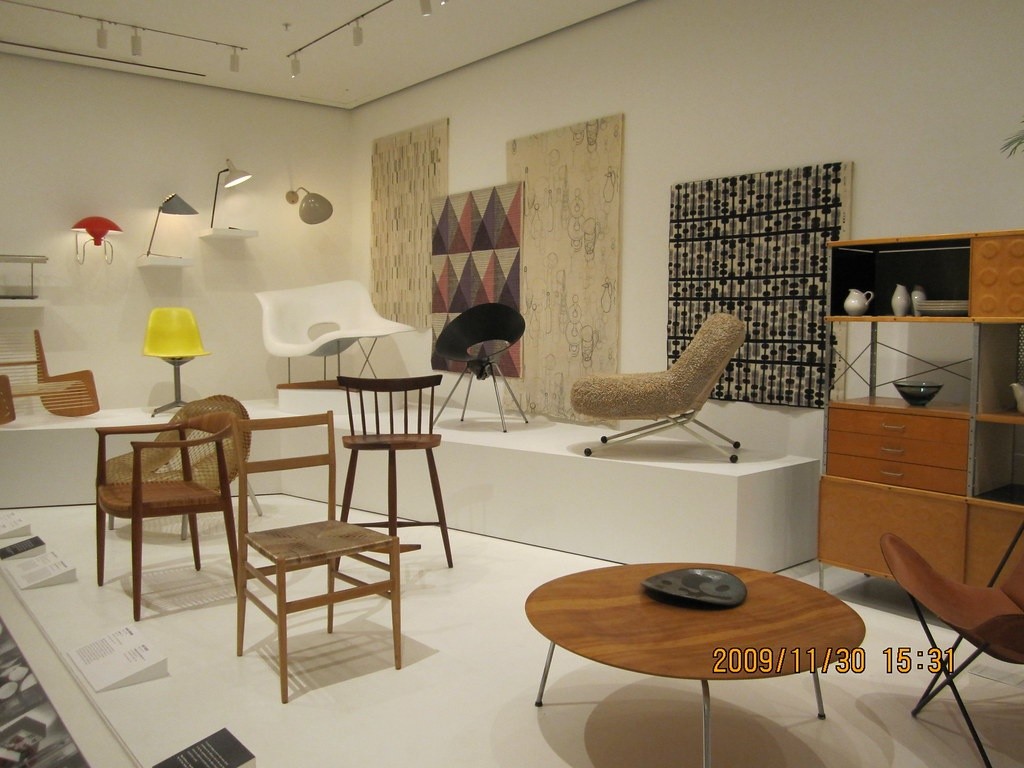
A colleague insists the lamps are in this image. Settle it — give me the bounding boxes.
[68,216,124,265]
[291,53,300,76]
[286,186,334,224]
[145,193,199,258]
[352,19,362,45]
[130,27,142,56]
[229,47,240,70]
[211,157,254,229]
[96,20,107,48]
[419,0,432,17]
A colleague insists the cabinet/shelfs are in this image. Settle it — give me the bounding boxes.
[816,231,1024,612]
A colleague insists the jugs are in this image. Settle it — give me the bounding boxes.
[844,289,874,316]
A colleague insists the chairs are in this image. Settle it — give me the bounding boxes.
[229,411,402,704]
[335,374,455,570]
[879,520,1024,768]
[430,303,529,432]
[104,394,263,542]
[0,328,100,427]
[573,307,745,462]
[258,280,418,381]
[142,306,214,415]
[94,410,239,621]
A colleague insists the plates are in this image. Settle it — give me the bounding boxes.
[642,568,746,606]
[916,300,969,316]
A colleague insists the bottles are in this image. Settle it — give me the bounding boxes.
[891,284,911,317]
[911,284,926,317]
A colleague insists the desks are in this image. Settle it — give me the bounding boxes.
[524,563,868,768]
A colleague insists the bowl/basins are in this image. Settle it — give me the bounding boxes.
[893,381,944,406]
